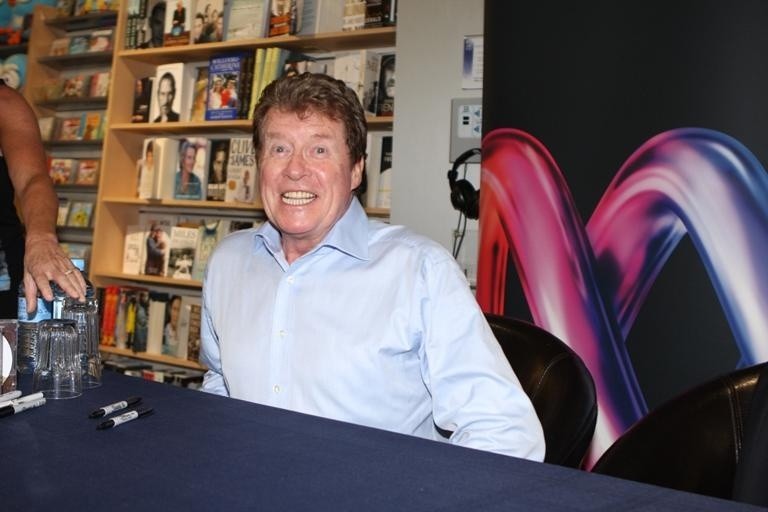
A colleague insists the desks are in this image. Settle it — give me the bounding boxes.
[1,354,768,512]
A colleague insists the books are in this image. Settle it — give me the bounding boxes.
[355,135,393,210]
[125,1,396,123]
[36,1,119,231]
[94,137,268,390]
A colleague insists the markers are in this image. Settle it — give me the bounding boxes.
[96,405,153,430]
[1,390,47,417]
[89,396,142,419]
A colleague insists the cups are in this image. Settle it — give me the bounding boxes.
[32,320,83,400]
[57,297,105,390]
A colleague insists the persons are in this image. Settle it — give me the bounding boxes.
[199,71,548,465]
[0,76,87,324]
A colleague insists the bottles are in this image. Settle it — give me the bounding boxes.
[0,319,18,394]
[17,273,53,375]
[53,259,95,318]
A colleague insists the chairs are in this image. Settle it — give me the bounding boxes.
[592,362,768,512]
[482,311,598,467]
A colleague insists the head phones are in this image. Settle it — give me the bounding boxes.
[448,148,482,220]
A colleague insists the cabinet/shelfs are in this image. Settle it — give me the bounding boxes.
[26,0,120,277]
[89,1,396,392]
